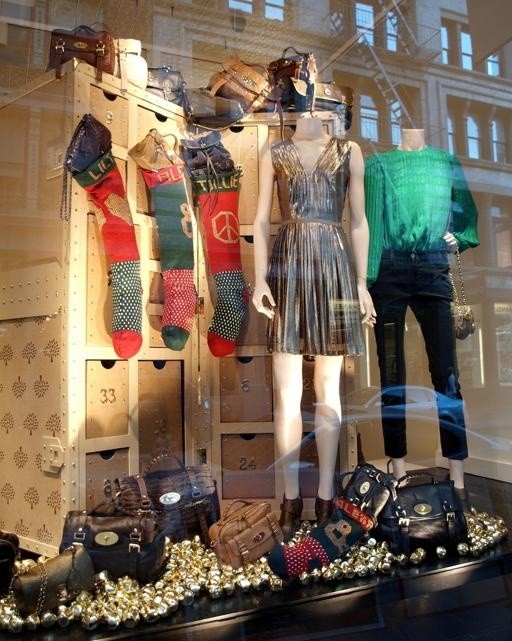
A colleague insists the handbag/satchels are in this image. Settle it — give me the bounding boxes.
[183,131,235,178]
[394,470,469,565]
[336,463,398,524]
[49,25,116,82]
[187,45,353,132]
[127,127,185,173]
[2,453,282,617]
[445,241,475,340]
[147,65,184,104]
[111,37,148,91]
[59,110,113,221]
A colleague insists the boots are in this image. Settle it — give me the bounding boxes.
[279,492,303,541]
[314,495,332,526]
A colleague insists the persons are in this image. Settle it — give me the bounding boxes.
[251,116,379,543]
[364,126,481,498]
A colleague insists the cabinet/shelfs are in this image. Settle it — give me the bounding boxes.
[0,59,357,559]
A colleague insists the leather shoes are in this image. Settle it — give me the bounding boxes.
[452,484,487,530]
